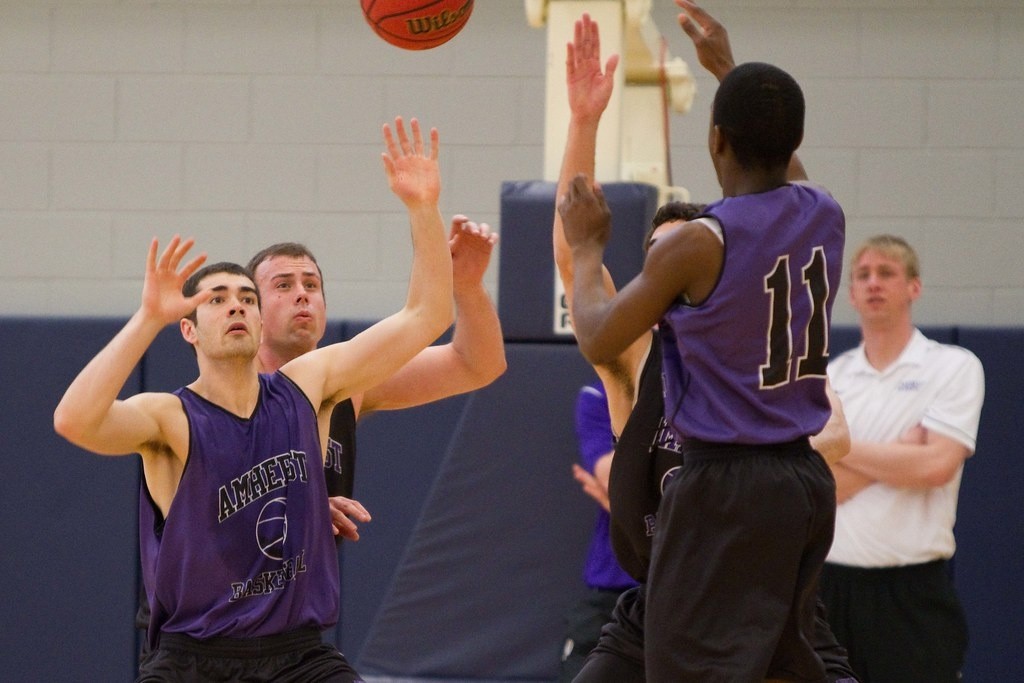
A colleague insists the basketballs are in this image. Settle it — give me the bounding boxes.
[359,0,474,51]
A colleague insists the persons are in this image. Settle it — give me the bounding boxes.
[556,0,986,683]
[246,214,509,649]
[53,115,458,683]
